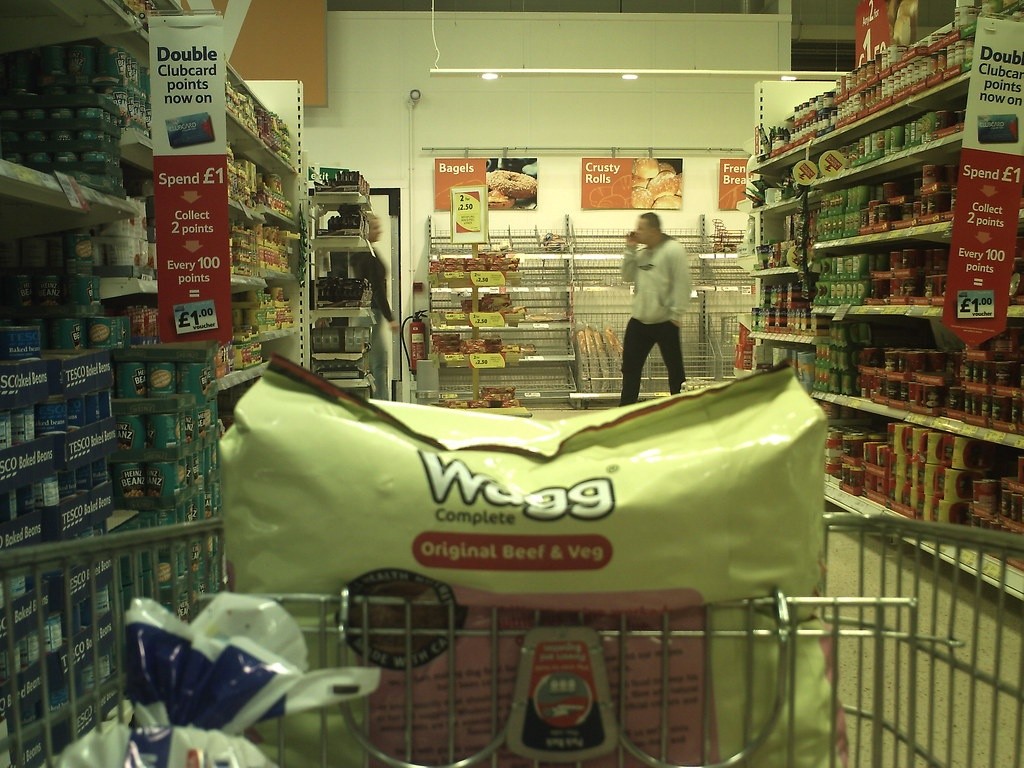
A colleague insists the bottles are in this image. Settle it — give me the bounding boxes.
[757,127,790,162]
[753,280,817,334]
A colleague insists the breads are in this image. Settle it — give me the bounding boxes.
[631,158,682,208]
[577,327,623,392]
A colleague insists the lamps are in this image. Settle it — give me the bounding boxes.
[429,69,853,81]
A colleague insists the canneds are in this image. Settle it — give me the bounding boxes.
[788,5,1024,536]
[1,41,222,734]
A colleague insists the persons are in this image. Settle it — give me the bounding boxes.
[350,213,398,398]
[621,212,691,405]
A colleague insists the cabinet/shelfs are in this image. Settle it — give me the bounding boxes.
[0,0,315,752]
[428,216,752,409]
[303,149,377,404]
[746,60,1024,595]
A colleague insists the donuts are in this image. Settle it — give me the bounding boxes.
[486,170,537,200]
